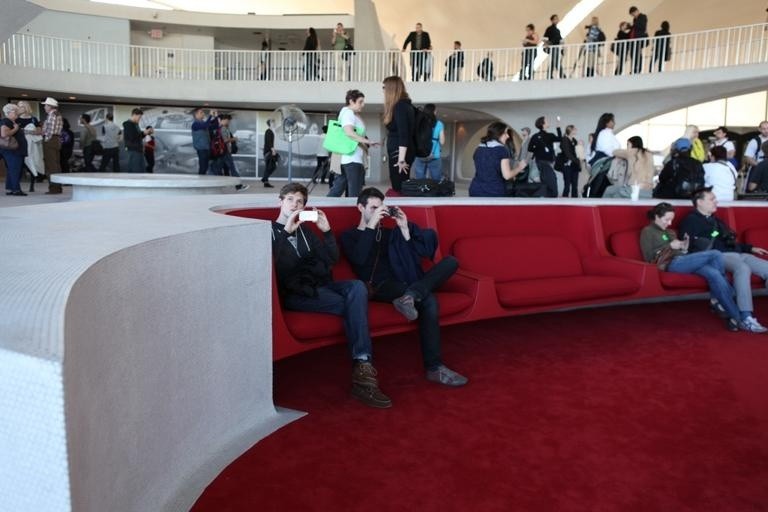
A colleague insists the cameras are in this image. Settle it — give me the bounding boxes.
[213,112,215,114]
[585,25,591,29]
[385,208,397,217]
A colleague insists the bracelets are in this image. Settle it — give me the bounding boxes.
[398,161,405,164]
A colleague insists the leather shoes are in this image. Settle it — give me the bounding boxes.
[350,364,377,388]
[725,317,739,332]
[708,299,729,318]
[350,386,391,408]
[263,183,273,187]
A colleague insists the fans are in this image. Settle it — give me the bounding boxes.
[269,104,309,184]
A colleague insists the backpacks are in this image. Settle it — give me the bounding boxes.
[671,158,704,198]
[412,106,436,158]
[210,126,225,157]
[590,27,605,46]
[606,158,626,185]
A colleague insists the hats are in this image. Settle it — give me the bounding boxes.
[40,97,59,108]
[674,138,692,152]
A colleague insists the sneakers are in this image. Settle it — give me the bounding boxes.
[426,365,468,386]
[738,317,767,333]
[393,294,418,321]
[237,184,249,192]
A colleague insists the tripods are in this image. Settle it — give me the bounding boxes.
[570,42,585,77]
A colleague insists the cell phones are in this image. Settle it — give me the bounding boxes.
[299,211,318,222]
[555,115,561,123]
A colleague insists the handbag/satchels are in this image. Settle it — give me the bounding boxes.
[0,135,19,150]
[656,247,673,272]
[400,178,455,197]
[322,119,365,156]
[641,32,649,49]
[365,281,377,298]
[610,39,626,55]
[505,183,548,198]
[734,168,748,195]
[342,44,354,59]
[665,45,672,62]
[514,164,528,183]
[92,140,104,155]
[554,153,578,174]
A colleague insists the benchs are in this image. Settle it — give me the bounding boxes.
[729,206,766,290]
[593,203,766,303]
[431,204,645,325]
[223,203,480,362]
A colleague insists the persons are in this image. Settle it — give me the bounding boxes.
[339,187,468,387]
[639,202,743,332]
[590,113,622,198]
[220,114,238,176]
[312,125,332,185]
[701,145,740,202]
[414,103,447,181]
[381,76,418,197]
[325,89,384,198]
[527,116,563,198]
[444,41,464,82]
[585,16,605,77]
[78,114,99,172]
[331,23,350,81]
[59,118,74,173]
[257,36,273,81]
[191,106,219,175]
[614,21,631,76]
[504,129,516,168]
[400,23,431,82]
[98,113,123,173]
[683,124,709,164]
[144,126,157,173]
[543,14,568,79]
[578,133,595,198]
[14,101,40,192]
[468,122,529,198]
[0,103,28,196]
[518,23,539,81]
[422,45,434,82]
[39,96,62,195]
[301,27,318,82]
[122,108,154,173]
[261,118,280,188]
[559,125,579,198]
[271,182,393,408]
[648,20,671,73]
[517,127,534,180]
[675,185,768,333]
[612,136,659,198]
[627,6,648,75]
[481,51,496,82]
[207,113,249,192]
[652,137,705,198]
[744,120,768,167]
[707,127,736,162]
[747,140,768,194]
